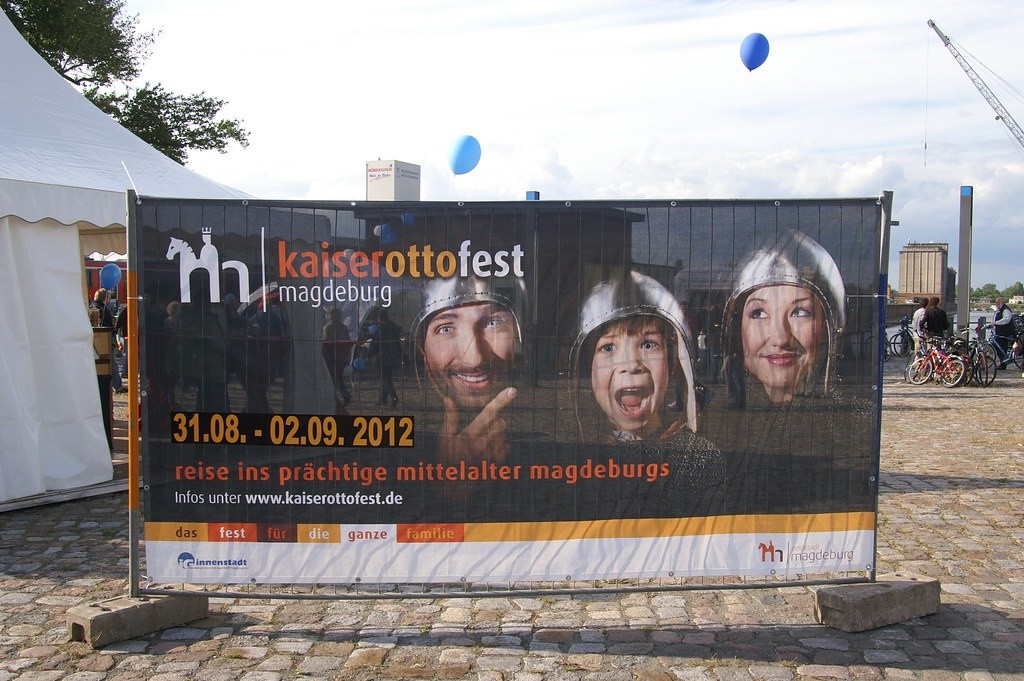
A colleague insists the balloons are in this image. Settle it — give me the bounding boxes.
[449,135,481,174]
[100,264,122,291]
[740,33,769,72]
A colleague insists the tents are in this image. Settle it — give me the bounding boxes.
[0,7,257,513]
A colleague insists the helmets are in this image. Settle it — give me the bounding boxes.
[413,264,530,397]
[568,267,699,445]
[721,227,845,398]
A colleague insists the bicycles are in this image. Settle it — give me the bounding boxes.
[865,314,1024,389]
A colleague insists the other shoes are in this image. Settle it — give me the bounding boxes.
[343,394,353,406]
[377,399,387,405]
[115,387,128,394]
[996,363,1007,370]
[392,398,399,409]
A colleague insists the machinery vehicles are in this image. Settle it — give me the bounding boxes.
[922,18,1023,160]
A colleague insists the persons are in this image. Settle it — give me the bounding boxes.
[912,297,949,378]
[90,288,128,393]
[145,228,876,525]
[989,298,1015,370]
